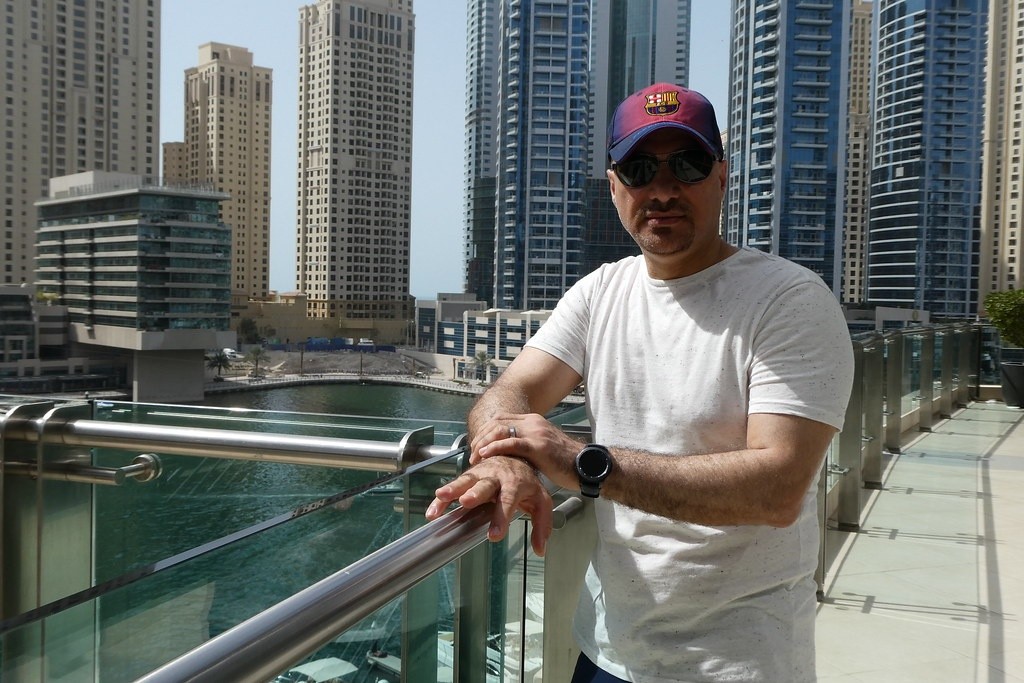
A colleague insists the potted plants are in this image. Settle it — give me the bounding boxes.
[981,289,1024,409]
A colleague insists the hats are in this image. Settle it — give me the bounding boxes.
[607,82,724,165]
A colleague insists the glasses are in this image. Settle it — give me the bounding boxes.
[611,147,722,189]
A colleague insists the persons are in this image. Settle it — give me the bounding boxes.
[423,82,855,683]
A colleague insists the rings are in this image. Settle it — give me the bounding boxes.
[509,426,516,438]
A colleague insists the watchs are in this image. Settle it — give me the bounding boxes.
[575,443,613,499]
